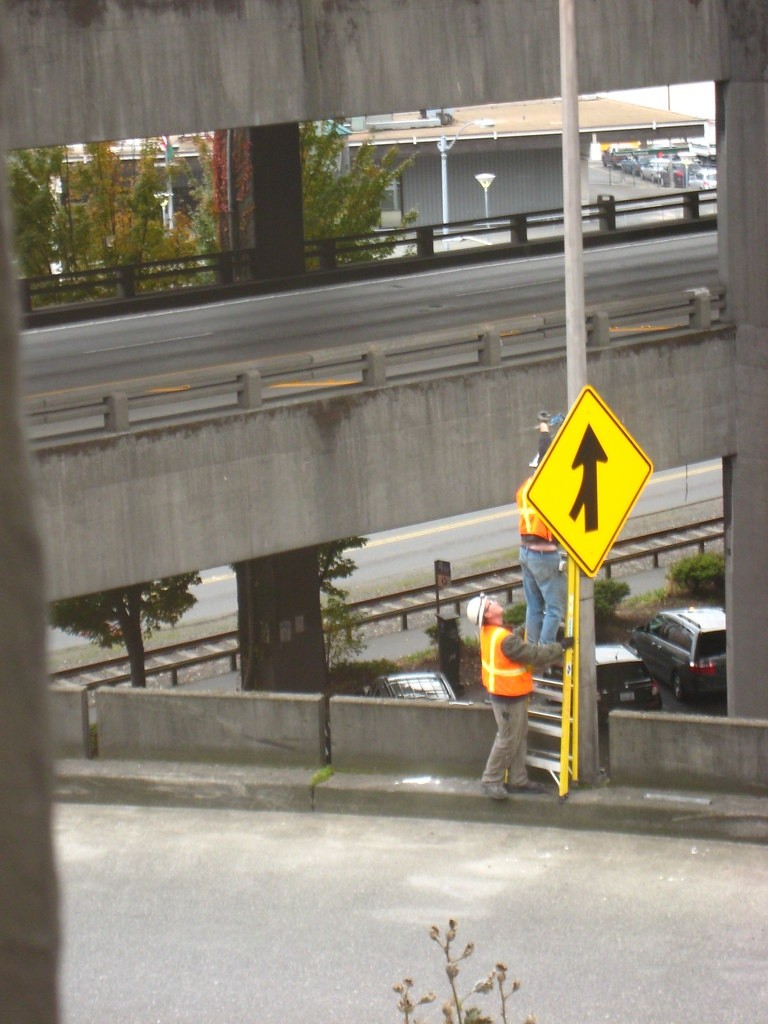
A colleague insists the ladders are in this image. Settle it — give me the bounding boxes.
[500,550,582,804]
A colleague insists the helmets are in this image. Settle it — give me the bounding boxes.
[466,591,488,627]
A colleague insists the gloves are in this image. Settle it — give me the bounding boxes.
[560,637,575,650]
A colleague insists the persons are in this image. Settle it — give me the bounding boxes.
[515,452,566,652]
[466,594,576,801]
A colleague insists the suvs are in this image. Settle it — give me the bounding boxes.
[629,605,726,702]
[542,642,663,733]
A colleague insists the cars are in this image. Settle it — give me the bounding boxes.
[598,129,719,191]
[367,672,464,705]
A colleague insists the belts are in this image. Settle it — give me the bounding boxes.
[520,544,558,556]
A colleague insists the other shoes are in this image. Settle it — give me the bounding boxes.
[482,780,509,801]
[507,779,546,796]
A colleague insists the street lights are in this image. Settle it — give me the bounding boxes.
[152,189,175,234]
[435,118,496,254]
[473,170,496,245]
